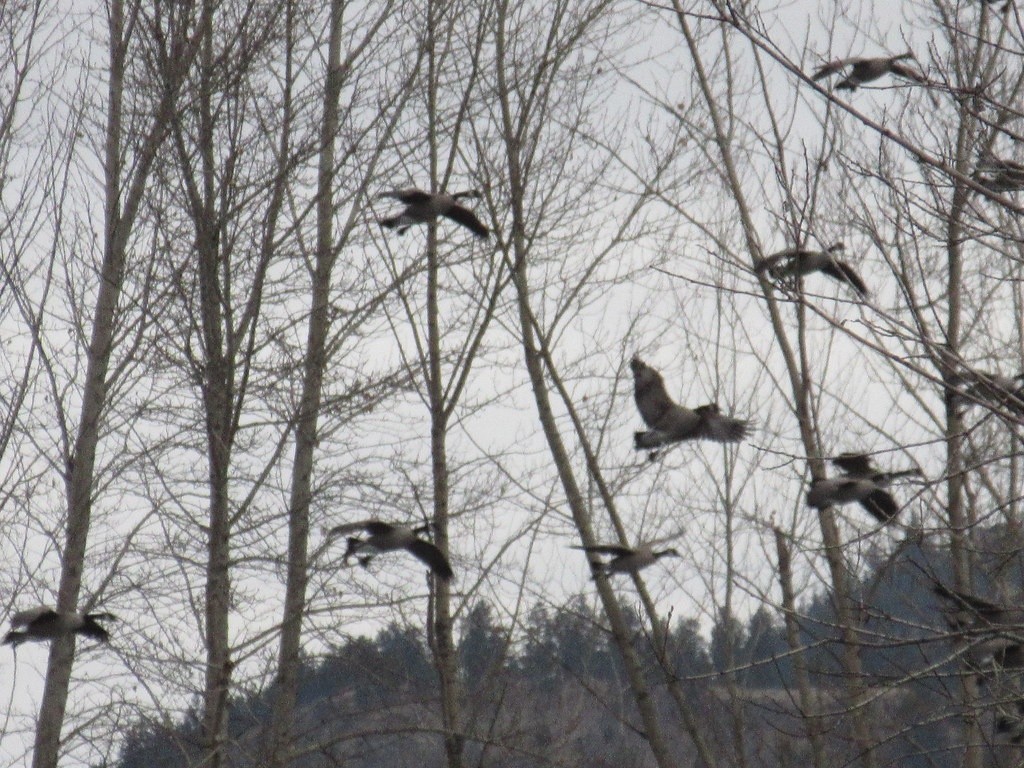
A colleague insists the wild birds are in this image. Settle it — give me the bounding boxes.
[810,49,924,94]
[748,241,870,298]
[804,451,929,524]
[933,580,1024,670]
[629,359,762,453]
[325,519,455,581]
[375,187,489,238]
[940,370,1024,420]
[563,526,687,586]
[0,607,117,648]
[968,144,1024,202]
